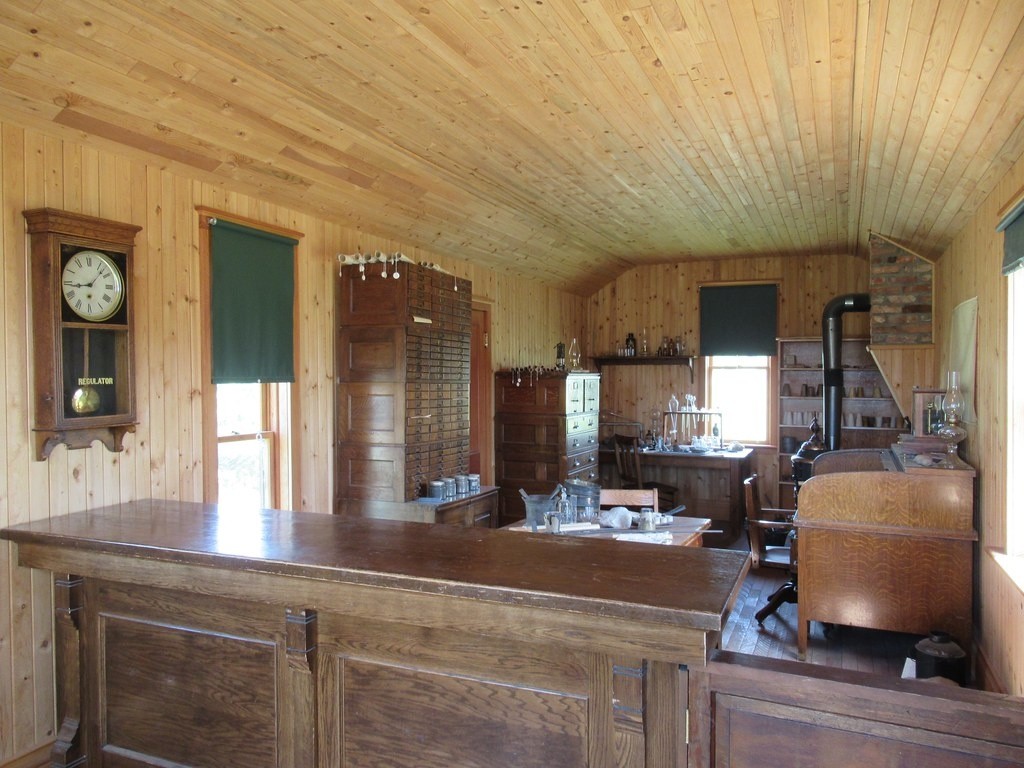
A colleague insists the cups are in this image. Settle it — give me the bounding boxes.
[817,384,823,397]
[808,387,815,397]
[872,387,882,398]
[857,387,864,397]
[782,437,796,452]
[848,387,856,398]
[638,508,656,531]
[543,512,559,531]
[801,384,807,397]
[783,384,790,396]
[656,516,673,524]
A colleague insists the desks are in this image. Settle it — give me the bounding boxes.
[600,443,753,539]
[496,511,713,548]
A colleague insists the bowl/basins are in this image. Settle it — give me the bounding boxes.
[565,479,601,522]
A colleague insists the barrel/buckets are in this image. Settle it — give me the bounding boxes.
[523,494,560,525]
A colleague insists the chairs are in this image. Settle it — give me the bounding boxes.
[599,487,659,514]
[743,472,795,574]
[612,434,679,518]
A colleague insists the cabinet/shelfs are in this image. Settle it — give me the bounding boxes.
[334,261,472,501]
[337,486,502,529]
[776,335,913,521]
[494,372,600,528]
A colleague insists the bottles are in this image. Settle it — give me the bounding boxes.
[676,336,682,355]
[669,339,674,356]
[558,488,572,524]
[641,326,650,356]
[626,333,636,356]
[663,337,668,356]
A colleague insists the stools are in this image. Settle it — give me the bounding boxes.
[755,530,836,640]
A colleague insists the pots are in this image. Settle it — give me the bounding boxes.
[908,632,971,683]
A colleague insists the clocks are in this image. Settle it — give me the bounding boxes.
[18,203,140,460]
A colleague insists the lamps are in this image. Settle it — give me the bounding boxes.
[569,339,583,371]
[640,326,649,354]
[934,370,969,469]
